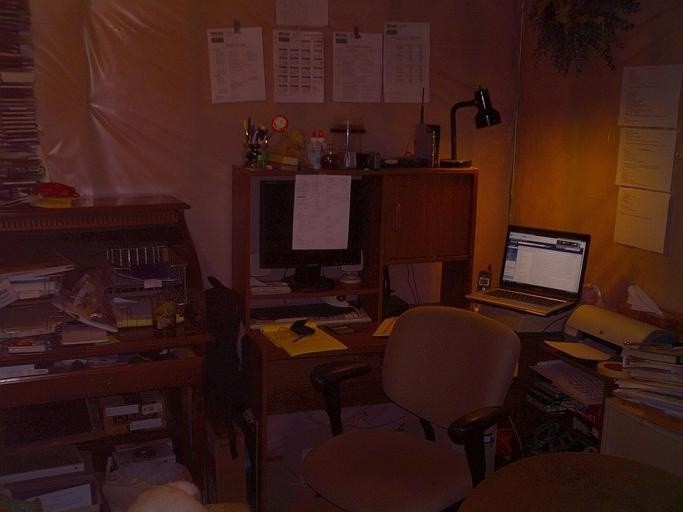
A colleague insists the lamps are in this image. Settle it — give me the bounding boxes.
[439,85,502,168]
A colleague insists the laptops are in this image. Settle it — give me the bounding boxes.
[465,224,591,318]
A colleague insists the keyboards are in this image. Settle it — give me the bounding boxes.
[251,300,372,325]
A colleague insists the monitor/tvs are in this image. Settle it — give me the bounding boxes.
[258,180,367,292]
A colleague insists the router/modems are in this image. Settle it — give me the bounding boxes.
[414,88,440,167]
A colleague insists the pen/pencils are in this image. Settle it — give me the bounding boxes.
[242,116,268,144]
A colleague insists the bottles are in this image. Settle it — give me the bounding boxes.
[311,127,342,171]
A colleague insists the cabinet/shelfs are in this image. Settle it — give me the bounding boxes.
[1,195,223,510]
[231,167,481,320]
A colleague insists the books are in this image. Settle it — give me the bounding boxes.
[613,344,683,419]
[0,265,152,353]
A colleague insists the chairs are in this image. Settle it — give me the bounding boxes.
[301,305,521,512]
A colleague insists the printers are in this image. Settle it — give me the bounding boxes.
[543,303,678,369]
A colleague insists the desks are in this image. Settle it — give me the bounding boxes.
[222,294,582,512]
